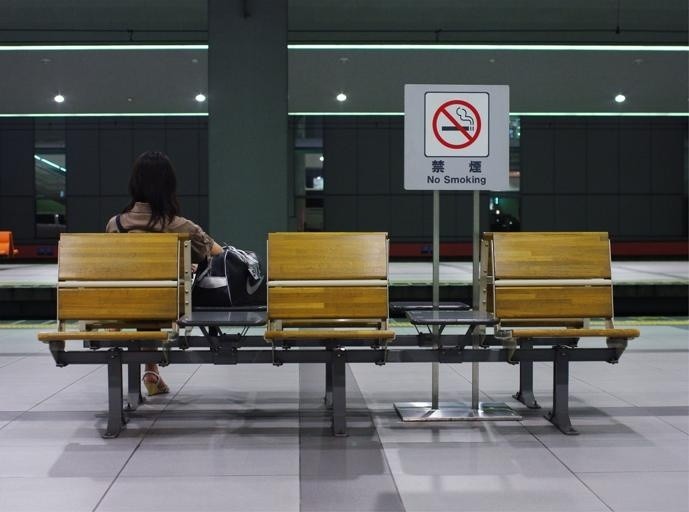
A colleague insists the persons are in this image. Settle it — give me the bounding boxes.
[106,150,225,397]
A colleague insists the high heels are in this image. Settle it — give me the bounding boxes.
[142,371,169,396]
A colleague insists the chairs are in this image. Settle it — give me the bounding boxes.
[479,231,584,408]
[266,233,395,437]
[38,233,180,437]
[0,231,19,256]
[491,231,639,435]
[76,232,208,412]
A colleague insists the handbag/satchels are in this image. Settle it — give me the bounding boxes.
[192,245,267,306]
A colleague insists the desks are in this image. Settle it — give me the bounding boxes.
[177,312,267,364]
[404,309,499,362]
[193,304,266,342]
[389,301,470,347]
